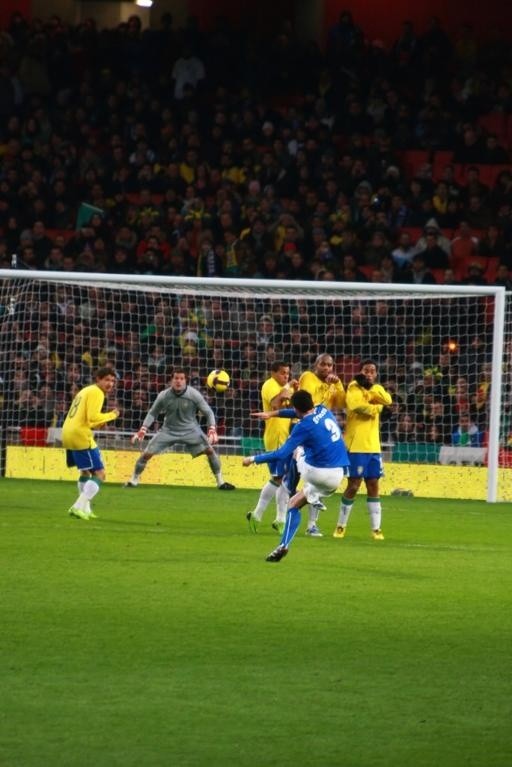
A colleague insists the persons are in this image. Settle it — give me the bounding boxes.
[246,360,299,536]
[333,359,396,540]
[62,367,120,519]
[250,391,349,561]
[1,14,512,448]
[290,353,348,537]
[121,369,236,490]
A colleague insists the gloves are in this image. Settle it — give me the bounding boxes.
[130,427,148,448]
[207,425,218,445]
[353,372,374,392]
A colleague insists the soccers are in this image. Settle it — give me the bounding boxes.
[207,370,230,393]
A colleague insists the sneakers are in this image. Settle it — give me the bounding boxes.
[305,526,323,537]
[266,544,289,562]
[315,502,326,511]
[333,523,346,538]
[217,482,235,490]
[271,520,285,536]
[126,476,138,487]
[369,527,385,540]
[246,510,260,535]
[68,506,99,520]
